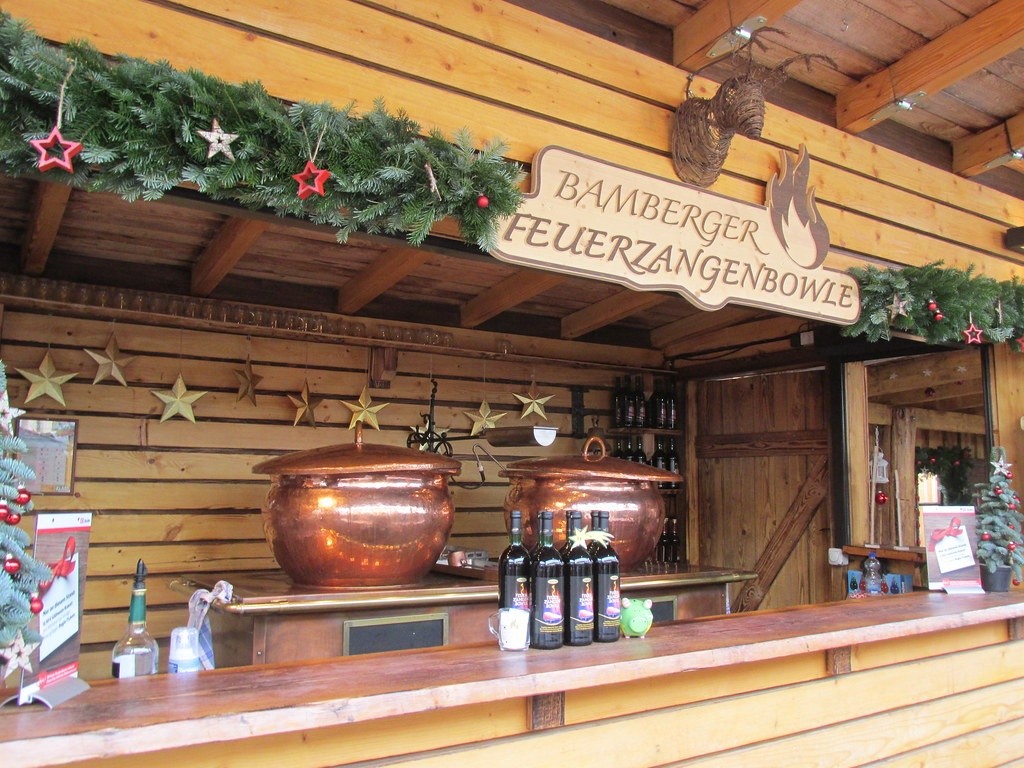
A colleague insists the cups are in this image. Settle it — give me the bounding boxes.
[487,608,532,650]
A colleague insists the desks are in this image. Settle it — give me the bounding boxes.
[0,588,1023,768]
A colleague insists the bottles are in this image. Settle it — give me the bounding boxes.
[864,553,881,596]
[530,508,620,646]
[605,374,685,564]
[111,559,159,677]
[168,627,200,674]
[498,509,533,613]
